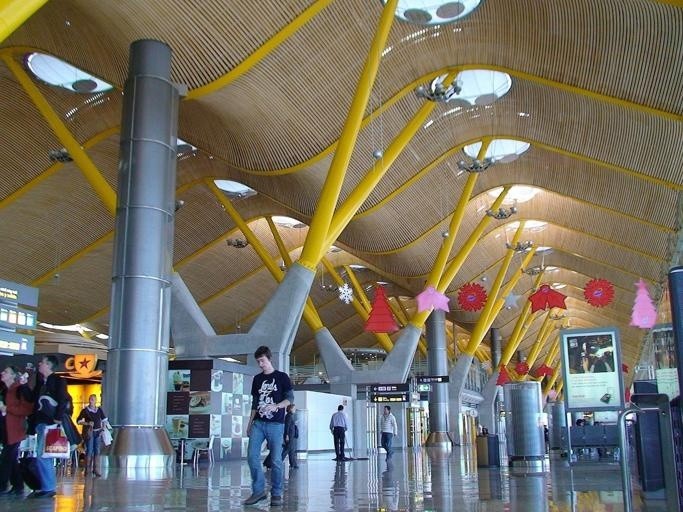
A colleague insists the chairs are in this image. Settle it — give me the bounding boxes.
[193,434,217,469]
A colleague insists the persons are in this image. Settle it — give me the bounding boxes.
[576,338,614,372]
[330,405,350,461]
[544,424,549,452]
[380,406,398,461]
[76,394,107,476]
[264,403,299,469]
[243,345,295,506]
[594,420,606,459]
[578,420,588,457]
[0,355,74,498]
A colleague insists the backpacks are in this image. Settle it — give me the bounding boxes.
[65,391,73,416]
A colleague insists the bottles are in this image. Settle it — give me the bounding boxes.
[258,402,274,419]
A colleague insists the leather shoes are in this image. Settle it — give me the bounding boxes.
[242,505,282,512]
[332,456,346,461]
[270,496,281,506]
[0,487,56,499]
[244,492,267,505]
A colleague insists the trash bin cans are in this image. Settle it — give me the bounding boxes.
[477,428,500,468]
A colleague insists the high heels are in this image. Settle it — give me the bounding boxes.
[83,470,102,476]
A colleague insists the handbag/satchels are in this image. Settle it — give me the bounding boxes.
[42,422,70,459]
[82,425,93,441]
[293,425,299,439]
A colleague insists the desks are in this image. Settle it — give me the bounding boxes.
[167,435,195,471]
